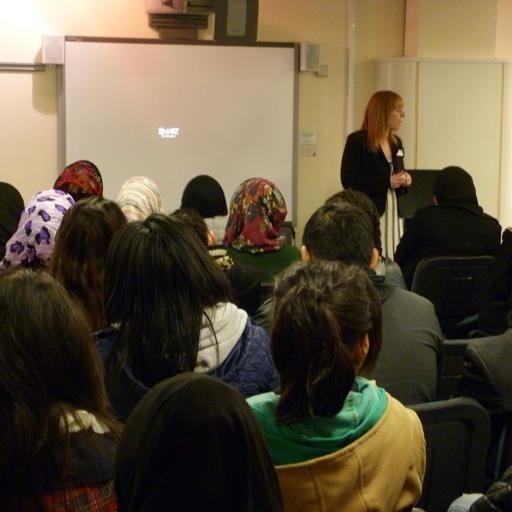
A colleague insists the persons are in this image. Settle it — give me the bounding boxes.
[0,158,512,512]
[338,90,413,261]
